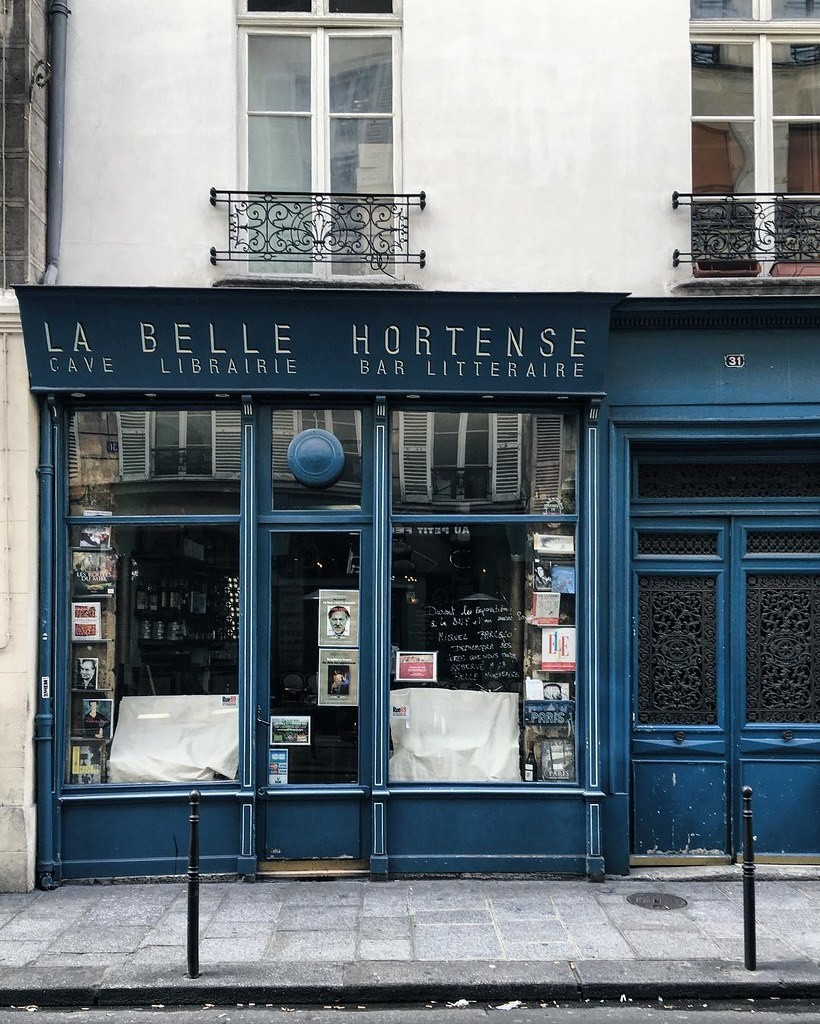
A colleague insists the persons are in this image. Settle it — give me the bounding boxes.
[535,565,551,588]
[332,668,346,691]
[328,607,350,636]
[78,659,96,689]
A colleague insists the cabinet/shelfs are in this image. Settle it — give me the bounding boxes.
[63,547,116,785]
[518,550,575,783]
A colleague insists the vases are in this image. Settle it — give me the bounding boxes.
[769,261,820,277]
[694,259,762,278]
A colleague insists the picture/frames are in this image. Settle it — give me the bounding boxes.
[270,715,311,745]
[318,649,360,707]
[318,589,360,648]
[396,651,437,683]
[268,749,288,785]
